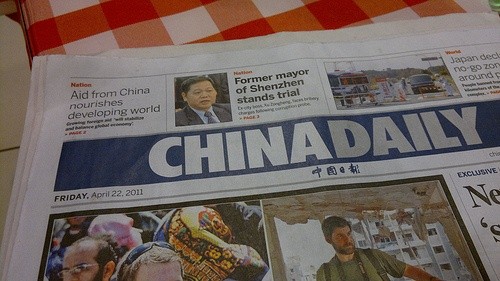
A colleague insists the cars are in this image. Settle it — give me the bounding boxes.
[407,74,438,95]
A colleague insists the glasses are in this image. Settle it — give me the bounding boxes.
[121,241,179,266]
[56,262,104,278]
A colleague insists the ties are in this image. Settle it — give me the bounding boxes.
[204,110,216,124]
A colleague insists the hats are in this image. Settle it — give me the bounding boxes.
[88,215,142,242]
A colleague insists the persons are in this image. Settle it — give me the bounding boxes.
[46,199,271,281]
[309,213,442,281]
[172,76,232,127]
